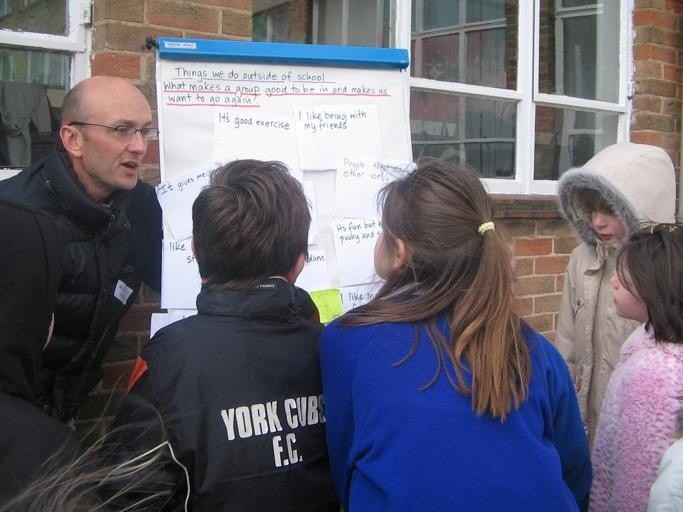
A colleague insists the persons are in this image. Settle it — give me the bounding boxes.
[0,76,164,425]
[550,140,678,449]
[646,405,682,512]
[589,219,682,511]
[96,160,341,512]
[319,158,592,512]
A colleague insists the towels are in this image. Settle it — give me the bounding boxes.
[1,49,70,89]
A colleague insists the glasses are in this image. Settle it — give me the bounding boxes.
[67,120,159,142]
